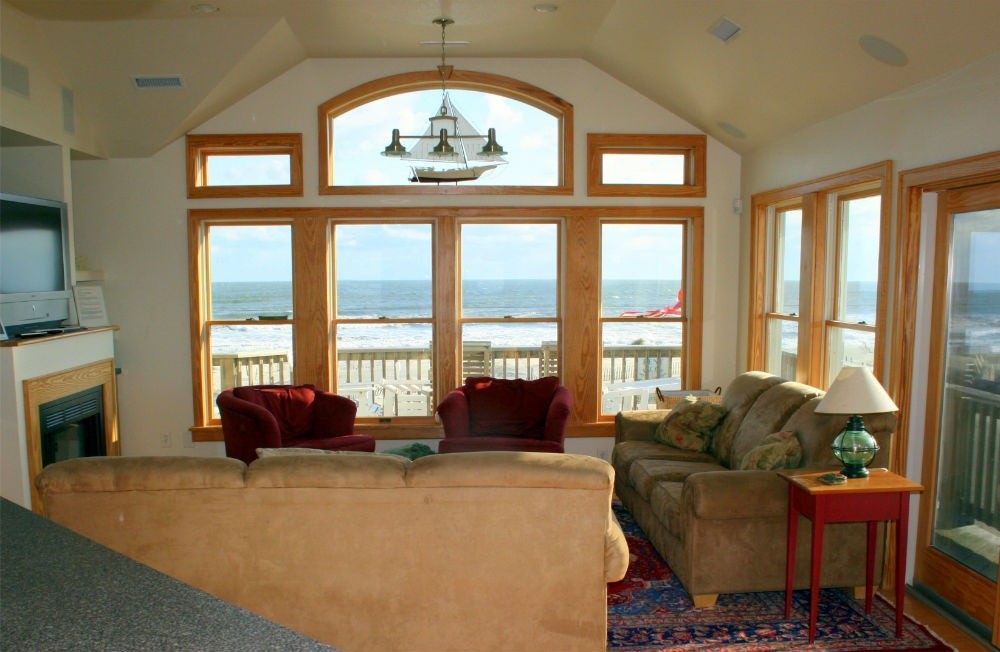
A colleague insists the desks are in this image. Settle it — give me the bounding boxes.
[775,468,925,644]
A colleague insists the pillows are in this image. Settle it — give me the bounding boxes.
[740,428,803,471]
[651,396,732,454]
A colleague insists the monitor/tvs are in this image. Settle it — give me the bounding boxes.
[0,192,82,338]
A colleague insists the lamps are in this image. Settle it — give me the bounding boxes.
[378,18,508,159]
[813,367,902,478]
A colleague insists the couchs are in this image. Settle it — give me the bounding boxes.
[436,376,573,456]
[37,451,629,652]
[216,383,376,466]
[613,367,898,608]
